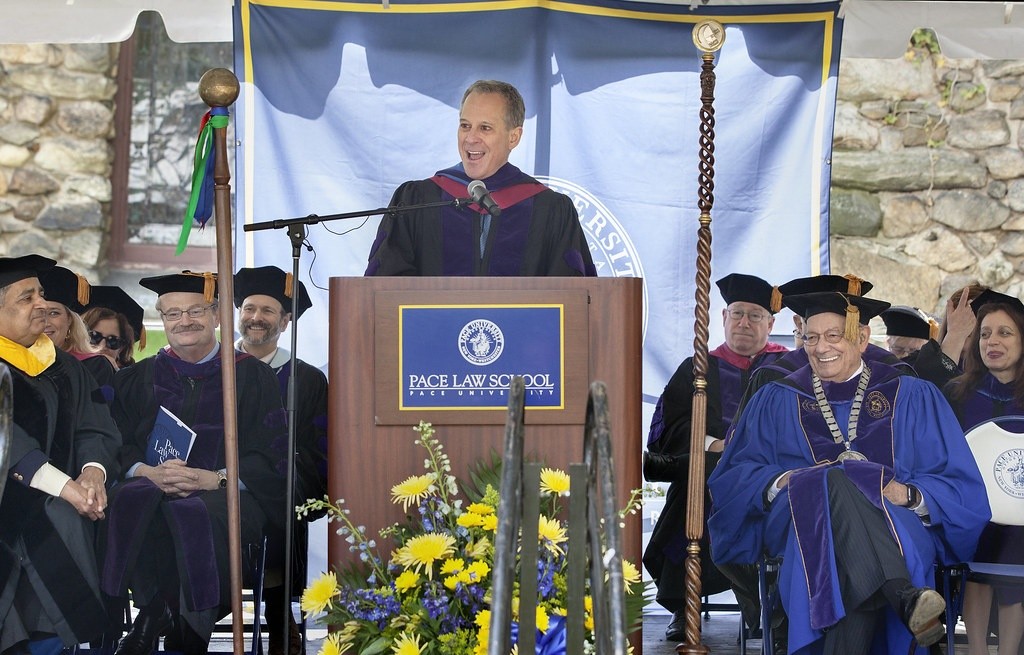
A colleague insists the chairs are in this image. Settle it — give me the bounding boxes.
[937,415,1024,655]
[62,537,307,655]
[699,559,962,655]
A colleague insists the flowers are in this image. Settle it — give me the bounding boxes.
[290,418,650,655]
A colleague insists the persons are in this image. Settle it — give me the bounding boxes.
[0,254,124,655]
[41,265,114,387]
[731,275,1024,655]
[703,291,991,654]
[364,78,597,276]
[97,270,289,655]
[643,274,790,643]
[233,266,328,654]
[80,286,146,371]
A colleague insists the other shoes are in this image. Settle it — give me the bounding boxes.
[263,593,303,655]
[26,631,65,655]
[90,627,123,655]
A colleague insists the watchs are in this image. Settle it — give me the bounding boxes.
[899,481,916,508]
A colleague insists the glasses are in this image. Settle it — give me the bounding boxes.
[727,309,766,322]
[889,344,918,357]
[88,330,124,351]
[161,303,216,320]
[801,329,846,347]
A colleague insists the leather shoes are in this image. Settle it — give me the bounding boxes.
[898,585,946,648]
[114,599,180,655]
[666,608,687,641]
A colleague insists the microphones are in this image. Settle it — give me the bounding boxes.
[467,180,501,216]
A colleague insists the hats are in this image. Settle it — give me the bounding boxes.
[233,266,312,321]
[970,288,1024,318]
[1,254,147,352]
[139,268,219,304]
[716,273,786,316]
[777,274,874,296]
[781,291,892,345]
[880,305,940,342]
